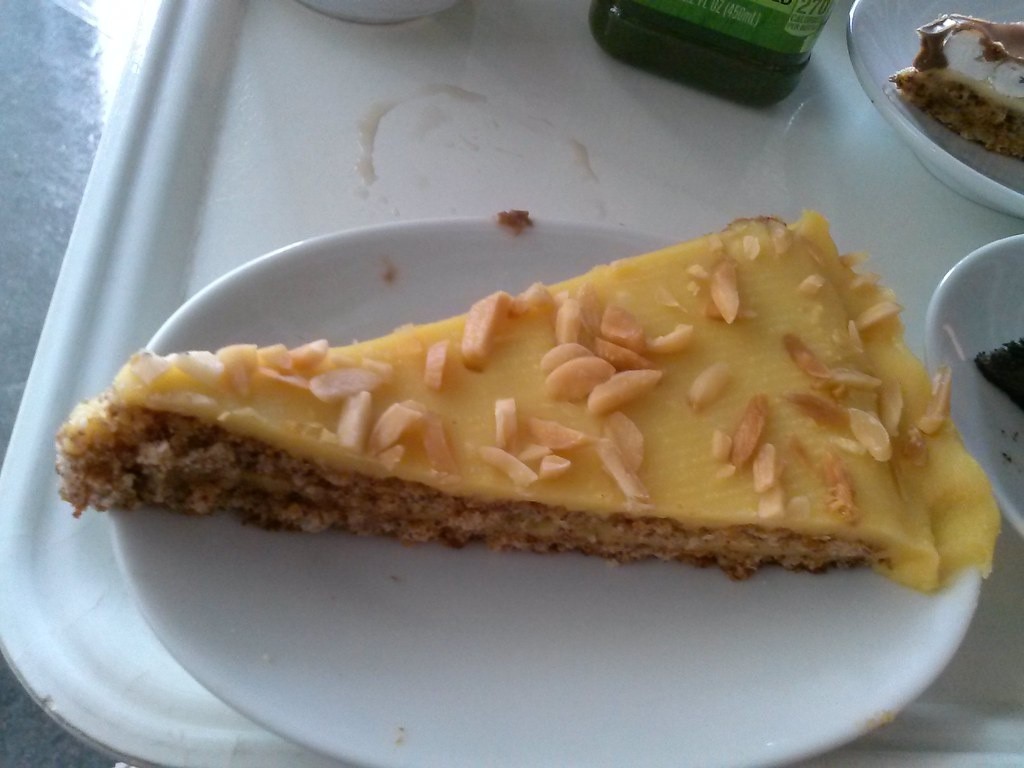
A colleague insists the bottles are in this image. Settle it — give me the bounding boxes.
[587,0,837,109]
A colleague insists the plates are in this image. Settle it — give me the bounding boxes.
[920,233,1024,543]
[846,0,1024,220]
[113,213,983,768]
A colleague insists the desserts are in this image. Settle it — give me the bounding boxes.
[888,15,1024,163]
[55,210,1001,594]
